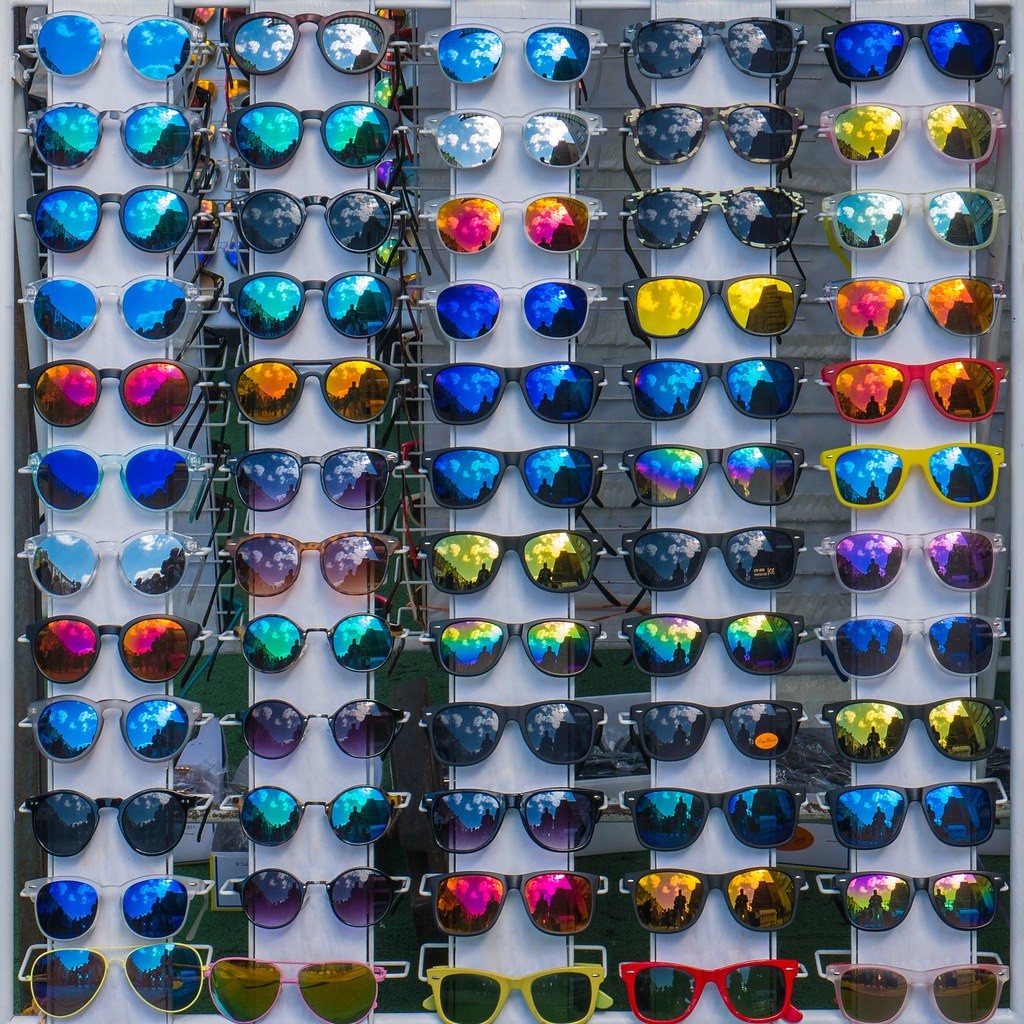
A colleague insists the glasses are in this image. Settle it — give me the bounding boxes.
[420,20,610,1023]
[617,19,808,1022]
[17,11,215,1022]
[814,20,1011,1022]
[205,10,416,1020]
